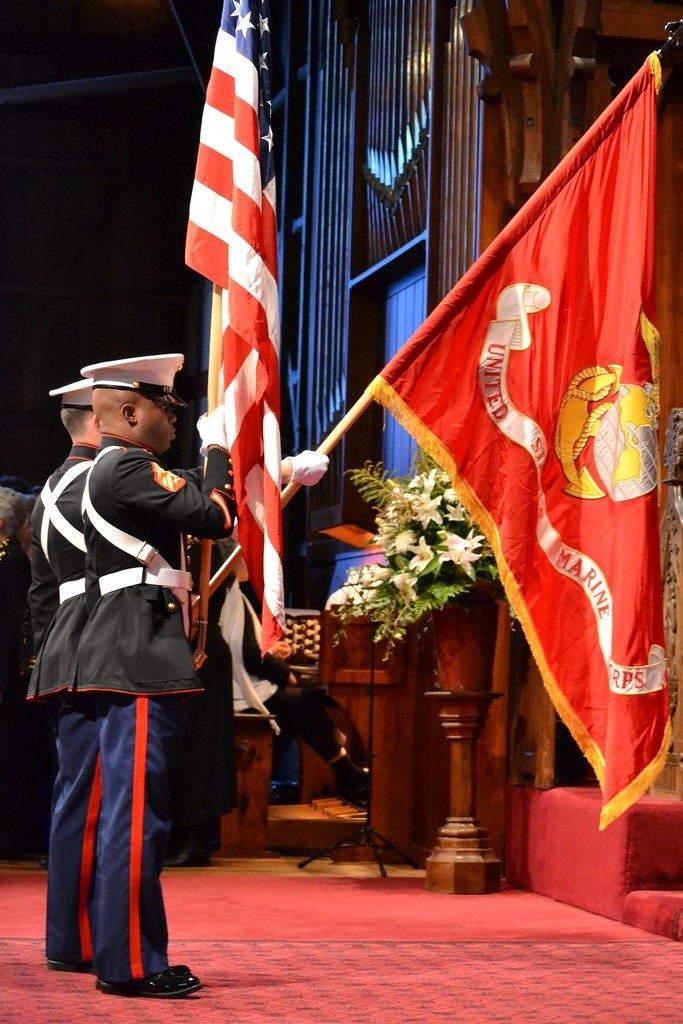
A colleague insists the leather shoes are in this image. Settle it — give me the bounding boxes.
[95,965,193,990]
[102,970,203,998]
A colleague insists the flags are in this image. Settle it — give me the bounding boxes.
[361,47,683,834]
[184,0,291,715]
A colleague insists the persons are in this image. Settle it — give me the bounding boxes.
[0,474,240,870]
[77,352,238,997]
[28,375,330,974]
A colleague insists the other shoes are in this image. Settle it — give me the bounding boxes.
[47,957,94,972]
[271,783,313,808]
[340,770,381,806]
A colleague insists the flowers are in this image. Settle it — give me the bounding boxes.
[332,444,519,660]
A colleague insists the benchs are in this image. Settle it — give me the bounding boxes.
[217,713,277,859]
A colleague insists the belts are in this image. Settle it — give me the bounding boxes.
[98,566,198,595]
[59,577,86,604]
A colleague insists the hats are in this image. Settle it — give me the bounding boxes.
[80,353,189,408]
[49,378,105,414]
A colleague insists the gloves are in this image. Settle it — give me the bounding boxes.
[196,405,232,458]
[292,449,332,489]
